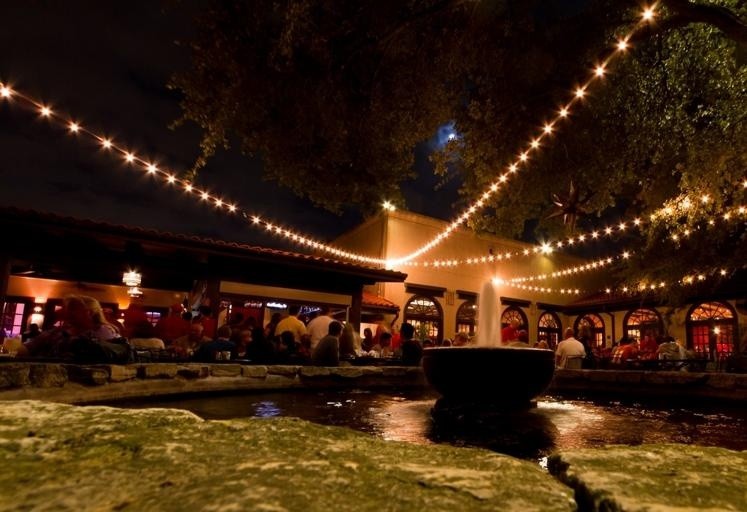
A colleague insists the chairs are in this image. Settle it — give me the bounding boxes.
[134,347,177,362]
[589,352,704,368]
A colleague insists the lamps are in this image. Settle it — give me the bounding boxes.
[122,271,143,298]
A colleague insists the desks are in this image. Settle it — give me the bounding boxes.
[358,356,393,364]
[233,357,252,364]
[0,352,16,363]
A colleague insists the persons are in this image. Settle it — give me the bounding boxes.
[22,293,122,356]
[224,309,312,367]
[309,321,344,367]
[126,319,167,350]
[173,322,212,351]
[339,317,469,367]
[516,329,548,350]
[555,327,588,369]
[273,303,309,353]
[21,322,40,341]
[500,317,521,342]
[190,324,238,363]
[305,303,340,357]
[609,334,690,373]
